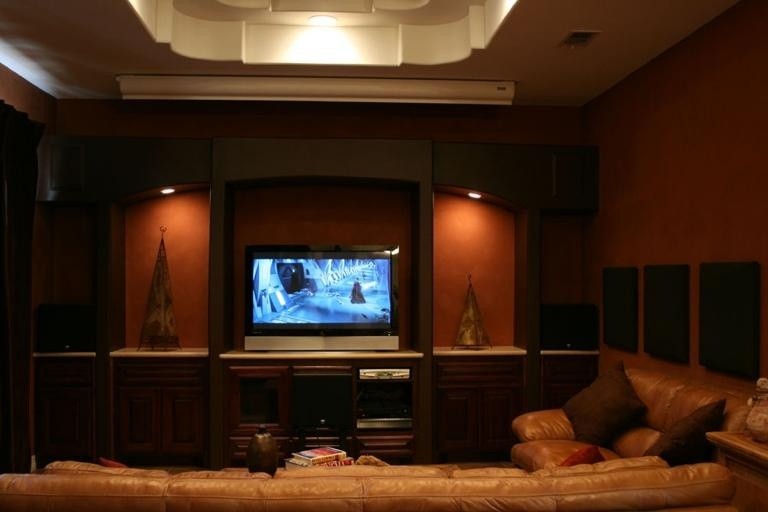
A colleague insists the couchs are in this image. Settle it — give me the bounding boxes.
[511,369,752,471]
[0,456,737,512]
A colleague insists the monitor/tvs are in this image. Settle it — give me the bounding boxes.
[241,242,403,352]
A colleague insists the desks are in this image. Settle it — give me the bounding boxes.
[705,431,768,512]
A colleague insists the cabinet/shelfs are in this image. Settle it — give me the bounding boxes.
[230,366,356,464]
[434,359,526,464]
[111,357,213,464]
[37,358,109,465]
[542,358,598,412]
[359,366,419,464]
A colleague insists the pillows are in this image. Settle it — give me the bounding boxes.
[562,360,646,446]
[560,446,606,466]
[643,398,727,465]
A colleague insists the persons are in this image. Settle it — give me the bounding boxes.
[348,276,366,304]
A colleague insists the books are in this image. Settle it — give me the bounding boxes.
[292,447,346,465]
[283,456,353,469]
